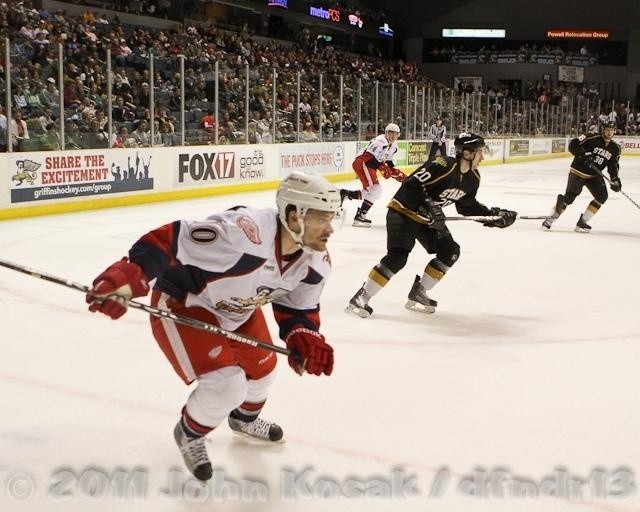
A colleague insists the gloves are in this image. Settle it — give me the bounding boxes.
[391,168,405,182]
[84,259,152,320]
[426,205,446,231]
[279,320,335,377]
[378,162,392,179]
[483,207,517,229]
[583,153,594,169]
[610,177,622,192]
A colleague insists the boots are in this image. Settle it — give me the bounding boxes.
[577,213,592,229]
[543,217,554,228]
[349,281,374,316]
[340,188,351,206]
[353,207,372,224]
[407,274,437,307]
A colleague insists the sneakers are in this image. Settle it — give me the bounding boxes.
[174,421,214,481]
[227,409,283,442]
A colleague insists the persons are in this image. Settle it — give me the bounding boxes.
[86,170,345,482]
[3,2,640,161]
[340,123,408,223]
[348,131,518,315]
[542,120,622,230]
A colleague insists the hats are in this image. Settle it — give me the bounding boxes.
[275,171,341,226]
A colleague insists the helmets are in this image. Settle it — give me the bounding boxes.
[385,123,401,137]
[455,132,485,159]
[601,121,616,131]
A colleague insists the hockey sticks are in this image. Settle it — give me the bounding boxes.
[388,198,558,223]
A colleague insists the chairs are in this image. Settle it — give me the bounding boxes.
[1,46,222,151]
